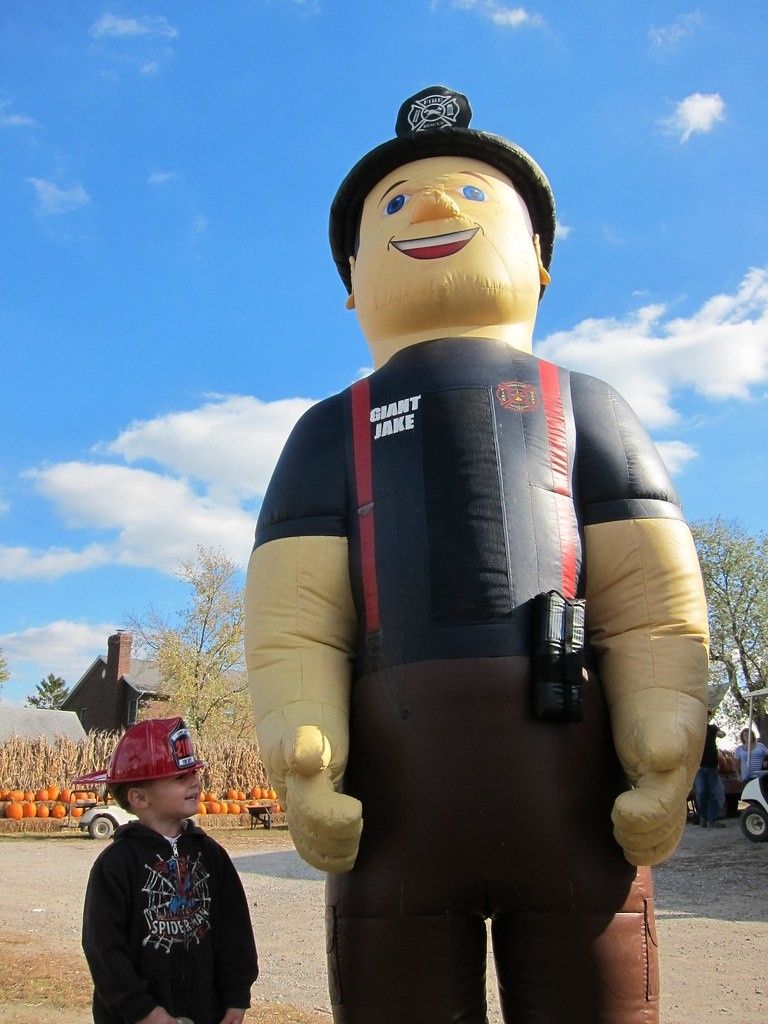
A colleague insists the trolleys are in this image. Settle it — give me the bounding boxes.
[246,804,276,829]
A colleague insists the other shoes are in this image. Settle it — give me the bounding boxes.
[700,819,707,827]
[707,820,725,828]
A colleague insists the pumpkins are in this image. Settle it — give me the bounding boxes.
[197,786,282,814]
[0,785,111,820]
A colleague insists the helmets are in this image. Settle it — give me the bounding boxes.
[73,717,206,782]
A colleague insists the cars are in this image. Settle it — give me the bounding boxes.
[75,804,198,839]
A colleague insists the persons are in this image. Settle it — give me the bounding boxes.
[732,728,768,787]
[79,717,260,1024]
[692,709,726,829]
[238,86,710,1023]
